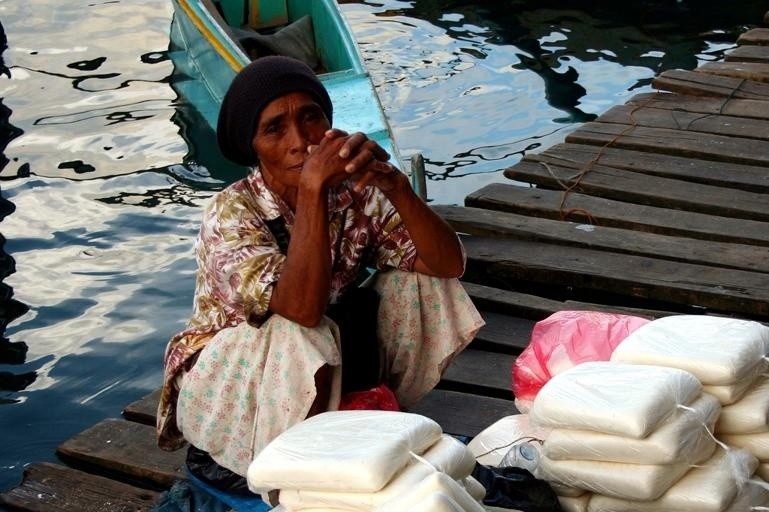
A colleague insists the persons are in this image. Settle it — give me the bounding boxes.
[156,56,487,507]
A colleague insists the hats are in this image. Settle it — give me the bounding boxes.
[217,56,333,167]
